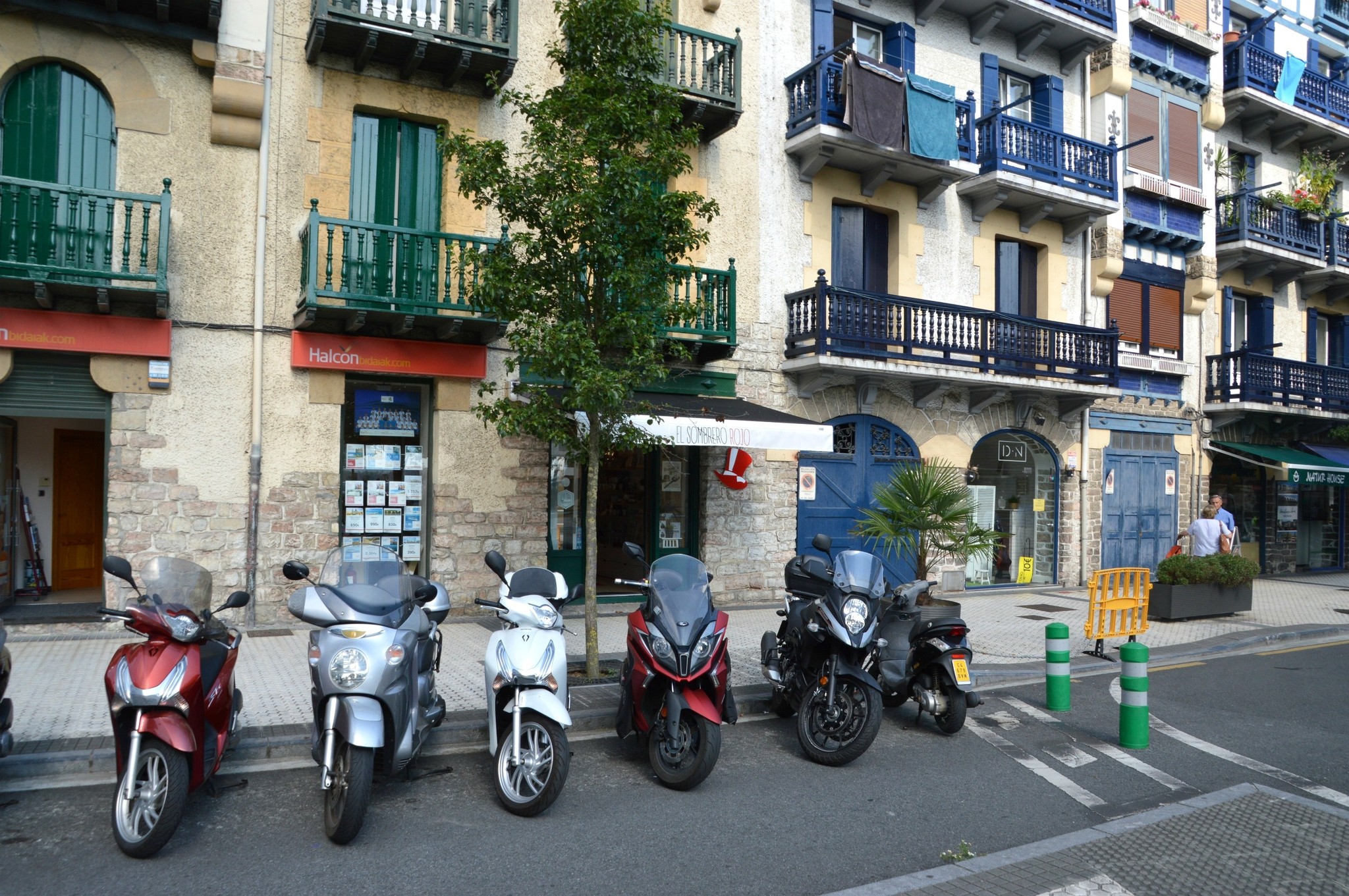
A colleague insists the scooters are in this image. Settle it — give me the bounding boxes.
[96,556,251,859]
[474,551,584,815]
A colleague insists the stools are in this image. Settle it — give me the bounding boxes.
[973,569,990,585]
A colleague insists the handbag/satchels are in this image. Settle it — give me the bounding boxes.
[1219,521,1229,554]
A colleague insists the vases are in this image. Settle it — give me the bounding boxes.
[1066,470,1073,477]
[1130,6,1219,56]
[1300,210,1320,222]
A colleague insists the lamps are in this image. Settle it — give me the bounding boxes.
[1009,405,1046,429]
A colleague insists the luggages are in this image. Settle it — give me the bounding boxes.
[1166,535,1184,559]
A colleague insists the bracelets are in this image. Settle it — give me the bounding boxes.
[1179,532,1185,537]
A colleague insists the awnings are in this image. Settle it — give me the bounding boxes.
[517,388,834,453]
[1301,442,1349,466]
[1207,440,1349,486]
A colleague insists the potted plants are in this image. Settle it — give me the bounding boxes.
[1008,496,1021,510]
[848,457,1016,622]
[1148,551,1261,622]
[1266,189,1285,211]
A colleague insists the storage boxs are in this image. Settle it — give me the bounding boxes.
[421,581,451,625]
[785,555,835,596]
[499,567,569,611]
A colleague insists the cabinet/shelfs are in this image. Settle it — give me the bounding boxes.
[596,468,646,582]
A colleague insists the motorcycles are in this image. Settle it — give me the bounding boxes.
[282,544,451,844]
[614,541,737,790]
[761,534,888,766]
[0,627,13,758]
[867,580,984,734]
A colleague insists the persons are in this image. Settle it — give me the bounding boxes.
[1176,505,1232,557]
[1210,495,1234,533]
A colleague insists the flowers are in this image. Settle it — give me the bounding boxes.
[1292,190,1324,214]
[1135,0,1221,41]
